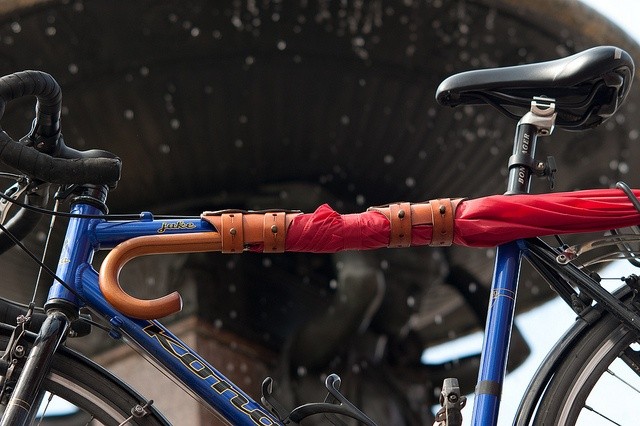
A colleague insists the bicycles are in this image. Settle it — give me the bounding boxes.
[0,44,640,425]
